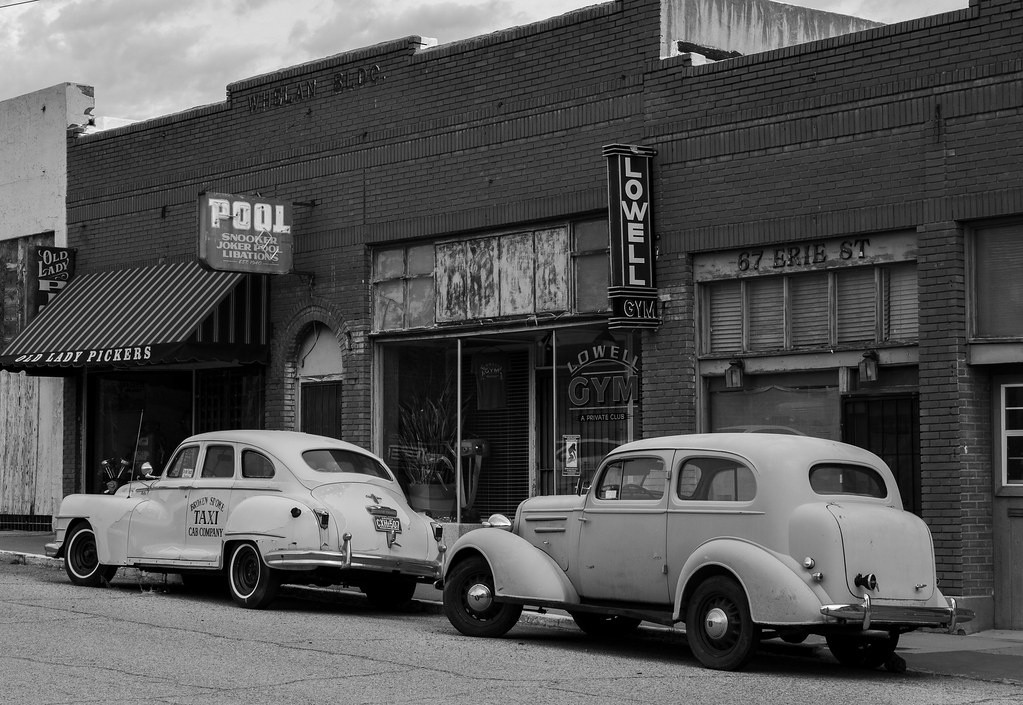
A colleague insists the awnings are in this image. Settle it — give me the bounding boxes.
[1,261,273,377]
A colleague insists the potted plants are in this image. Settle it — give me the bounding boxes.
[398,369,472,518]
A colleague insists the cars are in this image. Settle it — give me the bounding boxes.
[436,432,976,677]
[42,429,445,616]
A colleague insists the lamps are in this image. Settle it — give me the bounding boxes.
[725,358,746,389]
[857,349,880,385]
